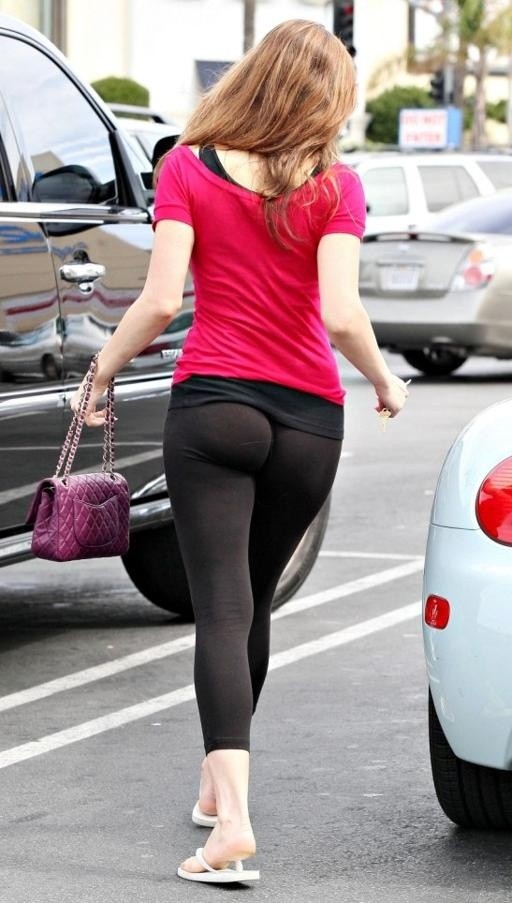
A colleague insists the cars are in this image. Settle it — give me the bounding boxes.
[423,399,511,831]
[341,152,512,375]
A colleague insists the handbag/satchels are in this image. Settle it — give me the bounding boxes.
[22,353,131,562]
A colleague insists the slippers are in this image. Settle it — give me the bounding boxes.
[176,847,261,883]
[190,801,218,827]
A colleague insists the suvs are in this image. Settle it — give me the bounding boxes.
[0,16,332,617]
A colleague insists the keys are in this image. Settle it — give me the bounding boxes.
[379,406,392,431]
[404,378,412,385]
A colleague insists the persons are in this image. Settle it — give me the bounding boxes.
[71,20,415,886]
